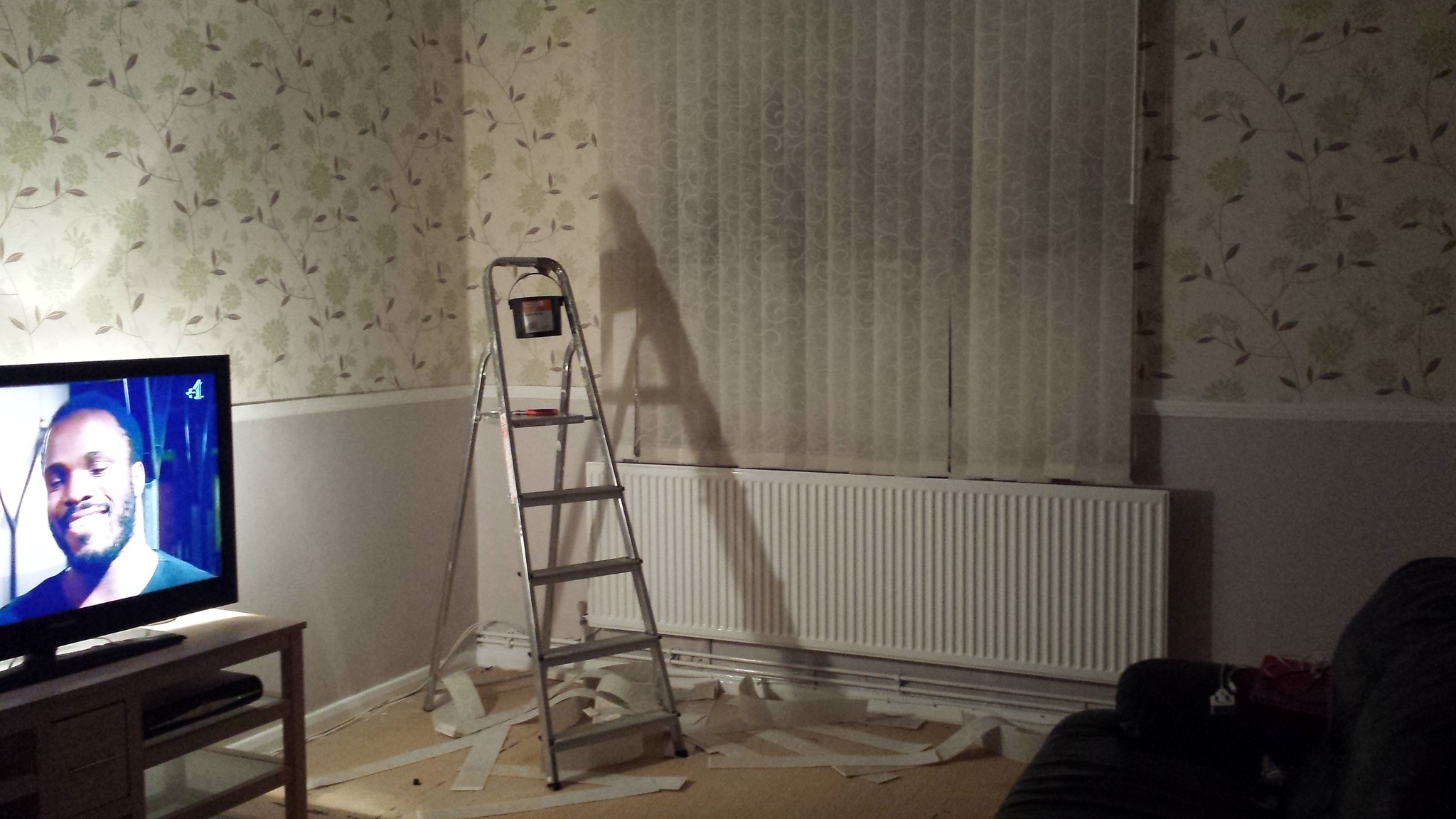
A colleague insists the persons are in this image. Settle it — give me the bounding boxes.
[0,391,219,626]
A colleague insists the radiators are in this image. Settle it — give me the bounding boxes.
[584,460,1172,685]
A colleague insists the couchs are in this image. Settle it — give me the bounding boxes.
[991,554,1455,819]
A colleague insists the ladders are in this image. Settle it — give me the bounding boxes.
[421,253,691,792]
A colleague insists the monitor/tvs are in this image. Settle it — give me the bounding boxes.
[0,355,240,692]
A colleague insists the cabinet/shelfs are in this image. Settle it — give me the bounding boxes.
[0,610,308,819]
